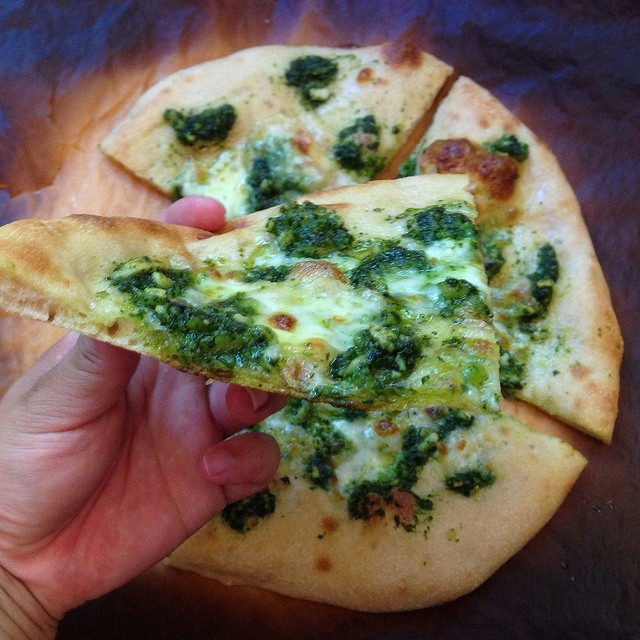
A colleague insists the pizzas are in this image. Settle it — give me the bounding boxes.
[0,41,624,613]
[0,173,506,416]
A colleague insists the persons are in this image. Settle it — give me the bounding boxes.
[1,194,292,640]
